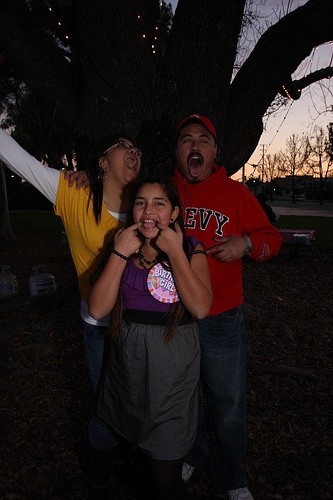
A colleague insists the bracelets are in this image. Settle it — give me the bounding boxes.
[110,248,129,261]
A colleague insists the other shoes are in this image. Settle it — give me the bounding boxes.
[181,462,195,480]
[229,487,254,500]
[86,447,112,488]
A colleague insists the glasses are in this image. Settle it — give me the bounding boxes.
[103,141,142,158]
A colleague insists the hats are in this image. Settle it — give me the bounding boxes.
[176,115,218,139]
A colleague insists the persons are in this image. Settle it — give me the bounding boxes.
[0,115,282,499]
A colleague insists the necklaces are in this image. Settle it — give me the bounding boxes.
[137,251,159,270]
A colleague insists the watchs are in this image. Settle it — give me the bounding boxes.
[243,234,252,257]
